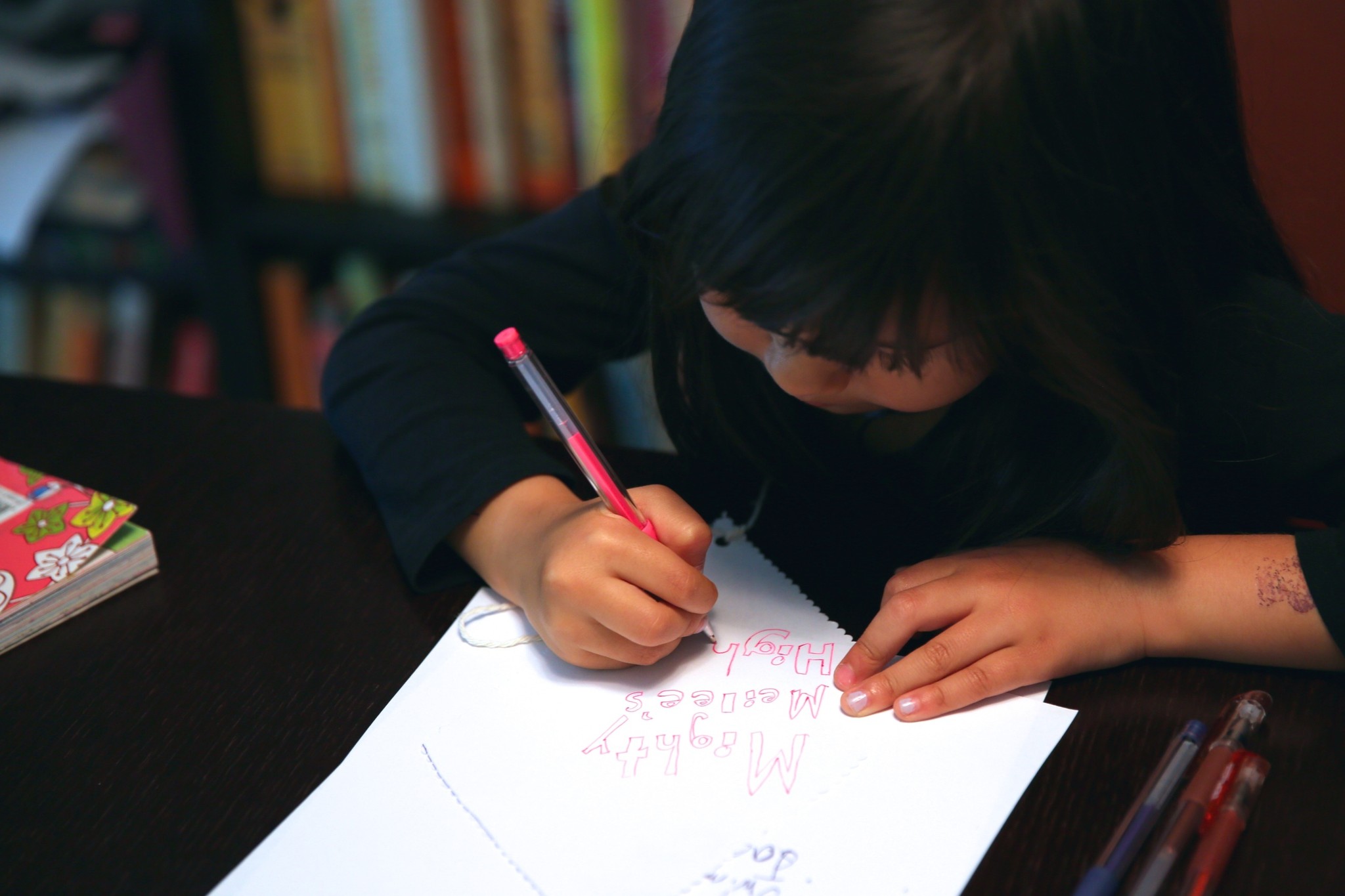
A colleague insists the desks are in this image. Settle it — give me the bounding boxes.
[0,372,1345,896]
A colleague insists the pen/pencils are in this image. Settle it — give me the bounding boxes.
[1184,748,1272,896]
[1075,719,1206,896]
[1133,689,1277,895]
[495,327,717,643]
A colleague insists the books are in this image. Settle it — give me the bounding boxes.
[0,1,676,455]
[0,458,158,659]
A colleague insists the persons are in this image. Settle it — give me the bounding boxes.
[322,0,1345,722]
